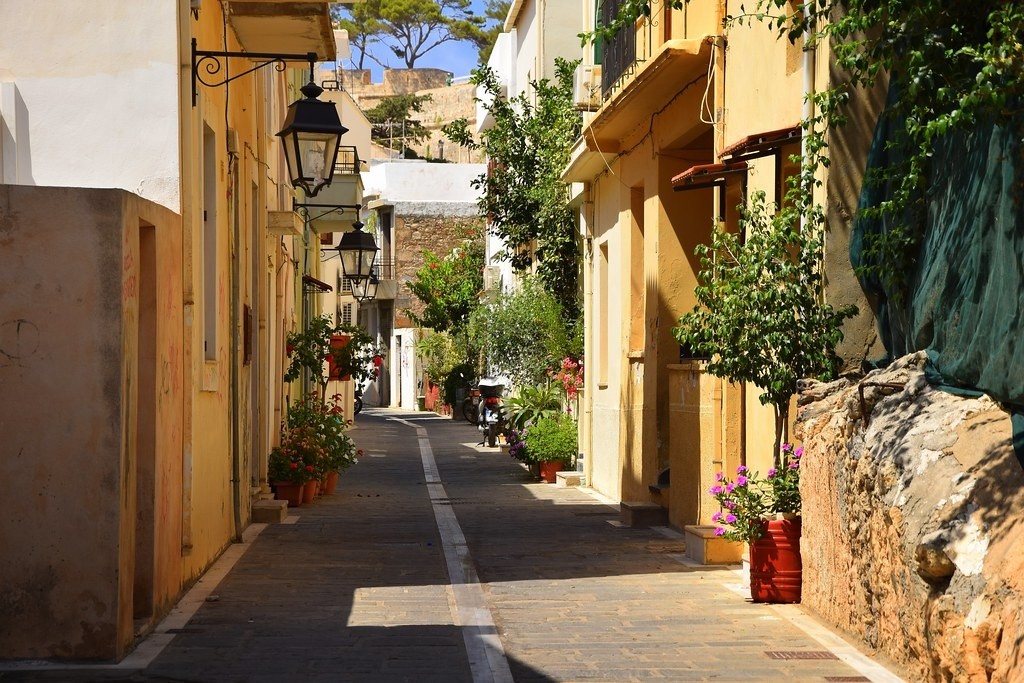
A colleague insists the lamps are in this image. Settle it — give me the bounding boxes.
[319,248,374,303]
[367,275,379,303]
[292,197,381,288]
[191,38,349,198]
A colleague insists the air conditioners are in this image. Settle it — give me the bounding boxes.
[338,264,357,336]
[483,266,500,300]
[573,64,602,111]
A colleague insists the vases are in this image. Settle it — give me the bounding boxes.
[273,464,342,506]
[748,515,801,603]
[532,461,539,474]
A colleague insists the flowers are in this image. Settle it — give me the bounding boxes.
[709,442,804,543]
[506,421,533,462]
[268,313,387,501]
[546,358,584,418]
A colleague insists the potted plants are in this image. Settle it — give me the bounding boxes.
[523,412,578,483]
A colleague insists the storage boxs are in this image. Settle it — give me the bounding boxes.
[478,376,505,397]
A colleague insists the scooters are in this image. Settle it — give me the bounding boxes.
[473,369,511,448]
[459,372,481,429]
